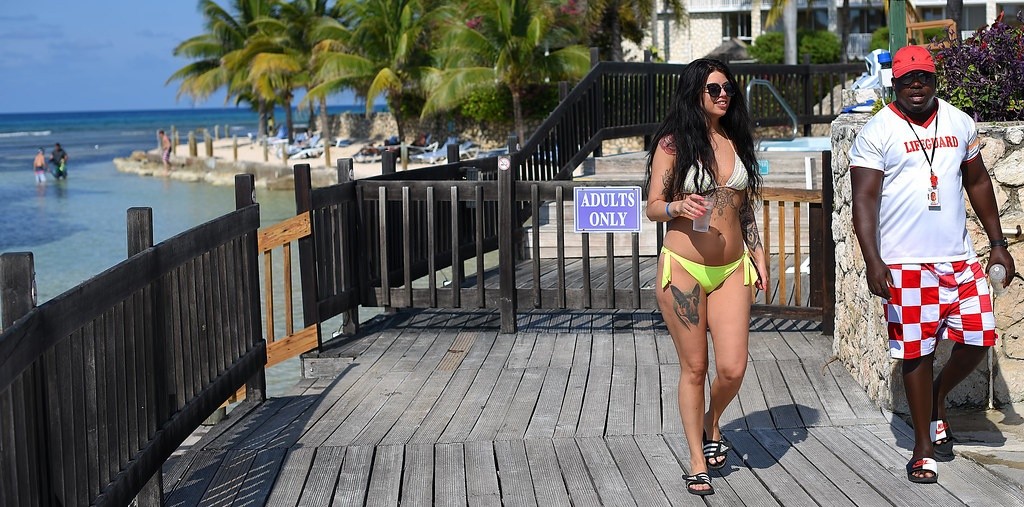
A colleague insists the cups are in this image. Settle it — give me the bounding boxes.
[692,196,715,232]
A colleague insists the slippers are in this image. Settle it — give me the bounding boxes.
[682,473,714,495]
[907,457,937,483]
[703,428,732,470]
[930,419,953,454]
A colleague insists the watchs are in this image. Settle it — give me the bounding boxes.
[990,236,1009,249]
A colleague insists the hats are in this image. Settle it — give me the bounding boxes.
[892,46,936,78]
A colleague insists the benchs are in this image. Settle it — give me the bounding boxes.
[883,0,959,55]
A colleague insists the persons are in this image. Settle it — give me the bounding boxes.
[646,57,770,494]
[33,142,68,181]
[647,45,663,63]
[354,130,426,157]
[848,45,1015,482]
[267,116,323,159]
[159,130,173,175]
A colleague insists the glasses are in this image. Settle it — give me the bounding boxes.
[900,72,935,85]
[704,83,735,97]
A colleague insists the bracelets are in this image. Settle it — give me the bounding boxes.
[666,201,676,219]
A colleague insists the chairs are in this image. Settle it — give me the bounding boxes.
[248,126,506,165]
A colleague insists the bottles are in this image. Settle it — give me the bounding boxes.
[989,263,1009,297]
